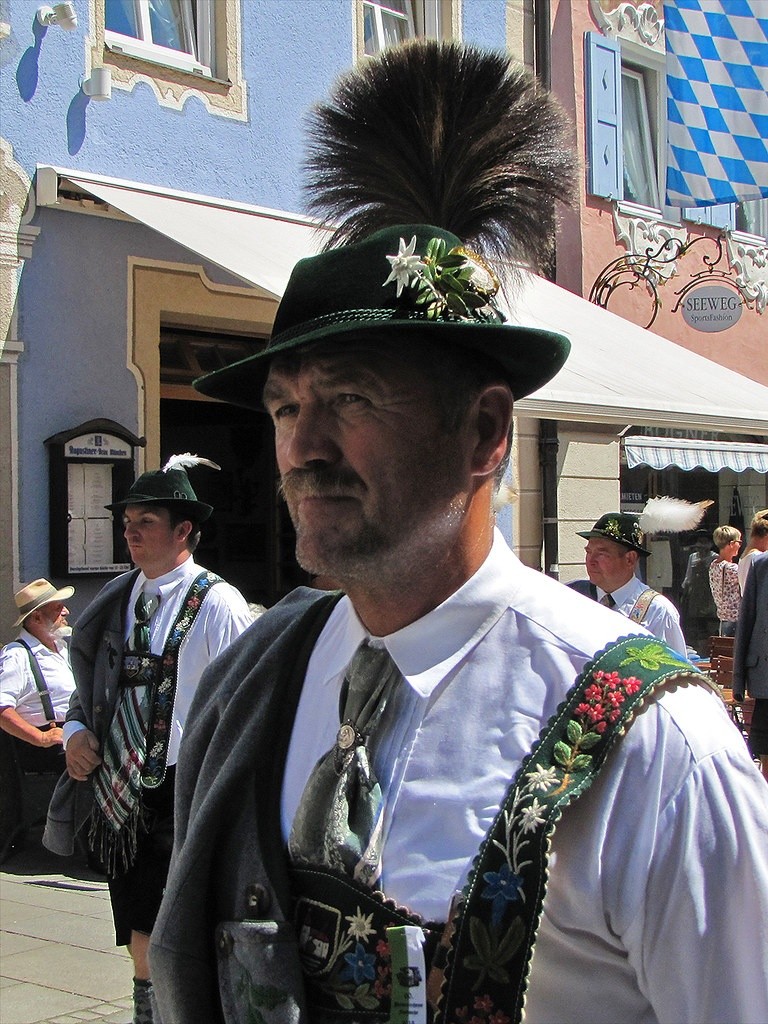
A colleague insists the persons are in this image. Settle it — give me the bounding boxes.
[679,530,720,636]
[58,450,256,1023]
[145,229,768,1024]
[564,513,689,660]
[0,577,83,768]
[731,550,768,780]
[738,508,768,596]
[709,525,743,637]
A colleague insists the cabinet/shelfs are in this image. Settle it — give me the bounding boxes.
[43,417,147,582]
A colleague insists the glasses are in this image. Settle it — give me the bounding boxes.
[728,540,742,545]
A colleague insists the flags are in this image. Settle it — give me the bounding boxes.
[662,0,767,207]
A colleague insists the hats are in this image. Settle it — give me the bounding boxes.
[188,223,572,414]
[104,468,214,525]
[10,577,75,628]
[575,511,653,557]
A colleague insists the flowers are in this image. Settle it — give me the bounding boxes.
[381,235,487,321]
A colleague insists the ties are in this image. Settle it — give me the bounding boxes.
[287,645,404,891]
[597,594,616,609]
[85,591,162,879]
